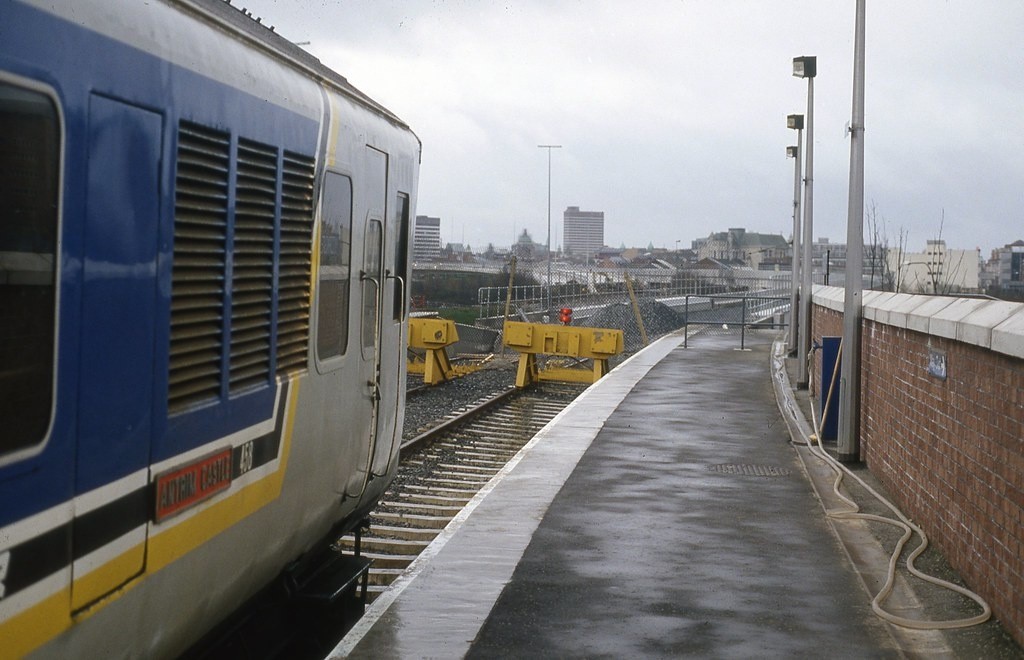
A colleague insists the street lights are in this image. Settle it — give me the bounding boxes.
[784,112,804,358]
[675,239,681,250]
[791,53,813,390]
[537,144,561,311]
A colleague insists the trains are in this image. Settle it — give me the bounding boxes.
[0,0,425,660]
